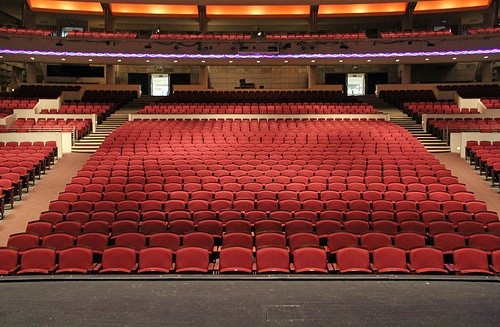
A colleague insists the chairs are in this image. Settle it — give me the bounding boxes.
[0,26,500,275]
